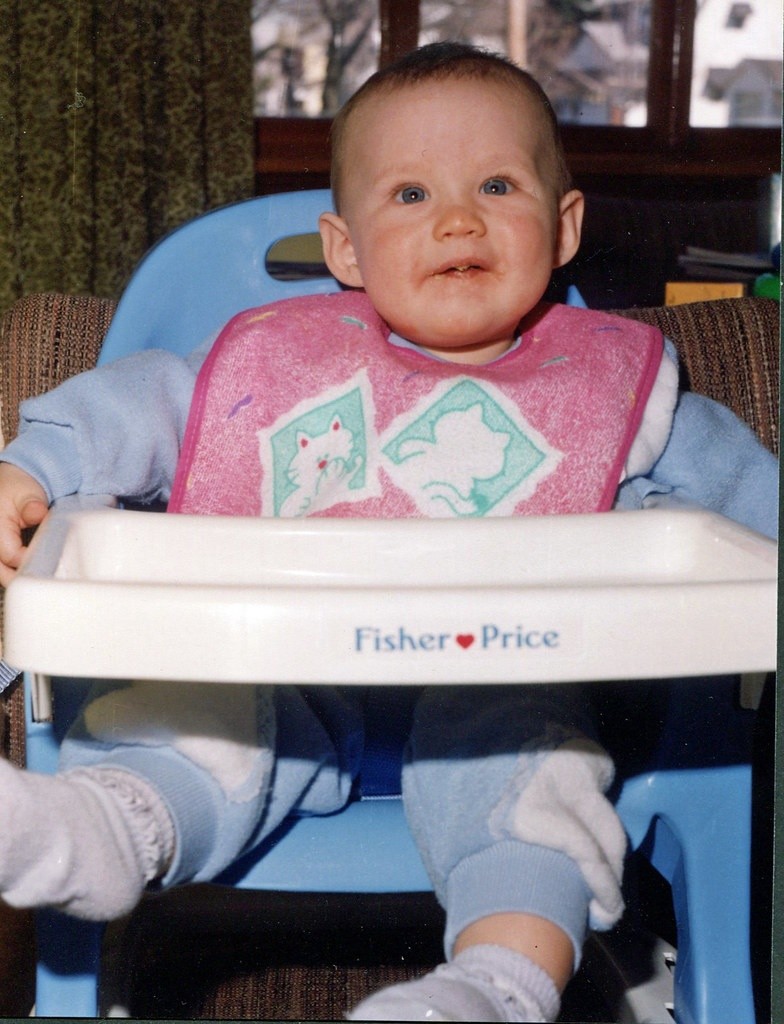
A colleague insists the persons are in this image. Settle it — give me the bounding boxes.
[0,44,781,1024]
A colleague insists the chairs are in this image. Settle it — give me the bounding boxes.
[4,188,784,1023]
